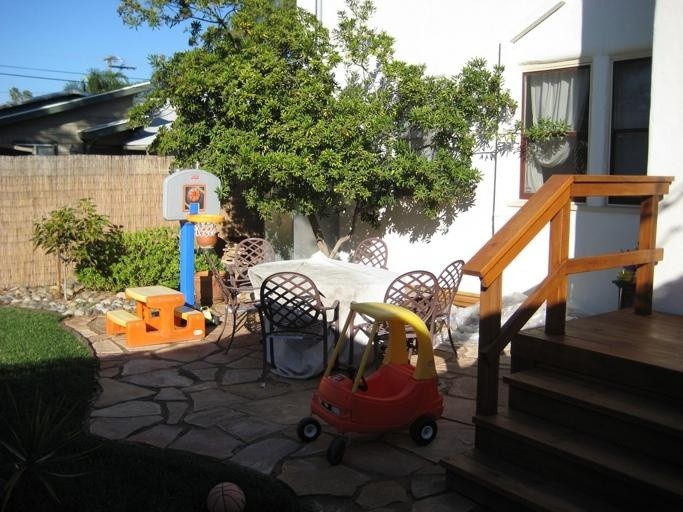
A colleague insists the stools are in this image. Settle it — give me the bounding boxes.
[103,306,205,346]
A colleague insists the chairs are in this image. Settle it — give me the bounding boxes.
[197,234,470,379]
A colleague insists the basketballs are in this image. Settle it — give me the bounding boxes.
[206,482,246,512]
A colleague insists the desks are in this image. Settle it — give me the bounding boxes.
[123,283,184,343]
